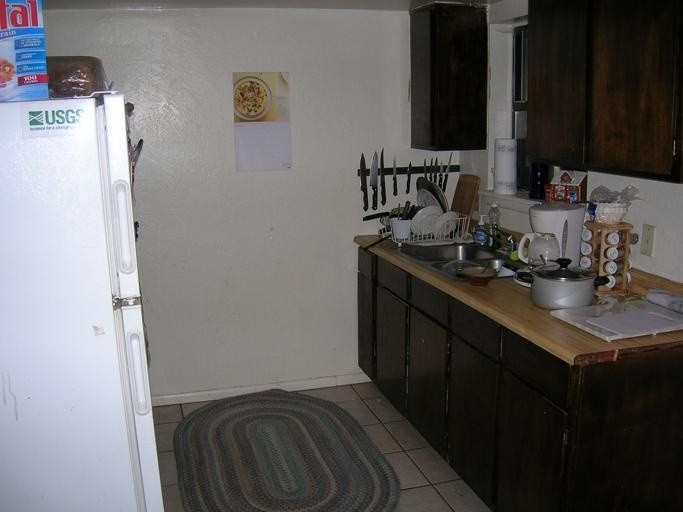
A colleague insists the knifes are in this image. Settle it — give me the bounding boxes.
[370,151,379,210]
[438,161,444,188]
[405,160,411,194]
[360,152,368,212]
[434,155,438,183]
[380,148,386,206]
[429,157,433,182]
[442,151,453,192]
[392,152,397,196]
[424,158,427,178]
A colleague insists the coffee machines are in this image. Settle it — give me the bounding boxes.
[529,202,585,271]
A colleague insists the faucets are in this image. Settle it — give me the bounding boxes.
[493,223,517,253]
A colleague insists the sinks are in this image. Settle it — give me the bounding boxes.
[428,258,519,282]
[388,240,496,264]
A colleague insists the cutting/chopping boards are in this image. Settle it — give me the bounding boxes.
[451,174,481,229]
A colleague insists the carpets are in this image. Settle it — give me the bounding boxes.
[173,388,401,512]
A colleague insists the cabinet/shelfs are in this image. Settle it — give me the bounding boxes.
[412,4,489,151]
[526,0,679,181]
[447,294,502,506]
[407,276,450,460]
[494,317,683,512]
[358,247,375,380]
[373,256,408,414]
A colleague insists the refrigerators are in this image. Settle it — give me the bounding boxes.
[1,93,167,512]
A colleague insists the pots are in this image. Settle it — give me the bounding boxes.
[512,258,610,311]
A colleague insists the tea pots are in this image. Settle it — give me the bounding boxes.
[518,232,560,266]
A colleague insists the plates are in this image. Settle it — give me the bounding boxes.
[433,210,458,239]
[410,206,443,235]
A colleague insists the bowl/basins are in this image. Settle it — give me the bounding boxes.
[462,267,495,287]
